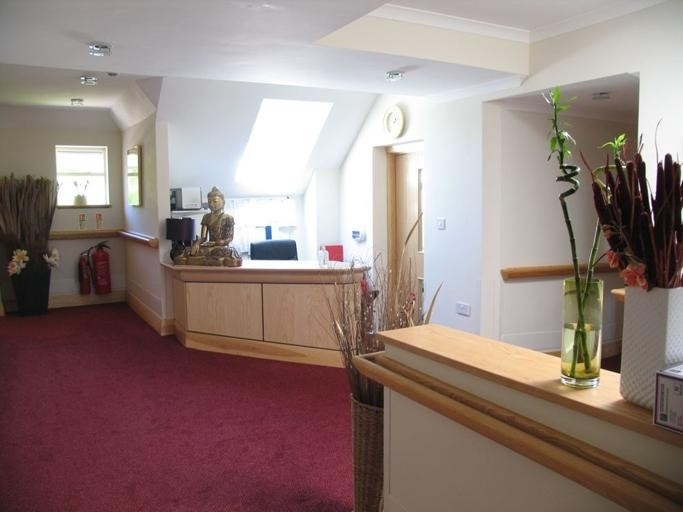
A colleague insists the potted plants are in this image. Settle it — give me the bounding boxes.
[537,87,628,390]
[577,118,683,412]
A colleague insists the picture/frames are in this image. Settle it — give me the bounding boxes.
[127,145,142,207]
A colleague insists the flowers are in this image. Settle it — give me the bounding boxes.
[7,248,60,278]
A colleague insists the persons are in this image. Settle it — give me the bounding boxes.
[170,185,244,268]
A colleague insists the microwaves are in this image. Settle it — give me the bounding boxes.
[169,187,203,210]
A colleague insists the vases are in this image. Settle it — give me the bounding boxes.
[12,270,51,315]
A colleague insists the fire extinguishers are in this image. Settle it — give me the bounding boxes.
[78,241,111,295]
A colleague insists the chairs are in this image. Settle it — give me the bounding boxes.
[250,242,297,260]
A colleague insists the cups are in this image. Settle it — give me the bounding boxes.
[79,214,105,230]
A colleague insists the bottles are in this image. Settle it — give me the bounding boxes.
[318,246,329,268]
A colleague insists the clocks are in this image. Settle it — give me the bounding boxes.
[383,105,404,139]
